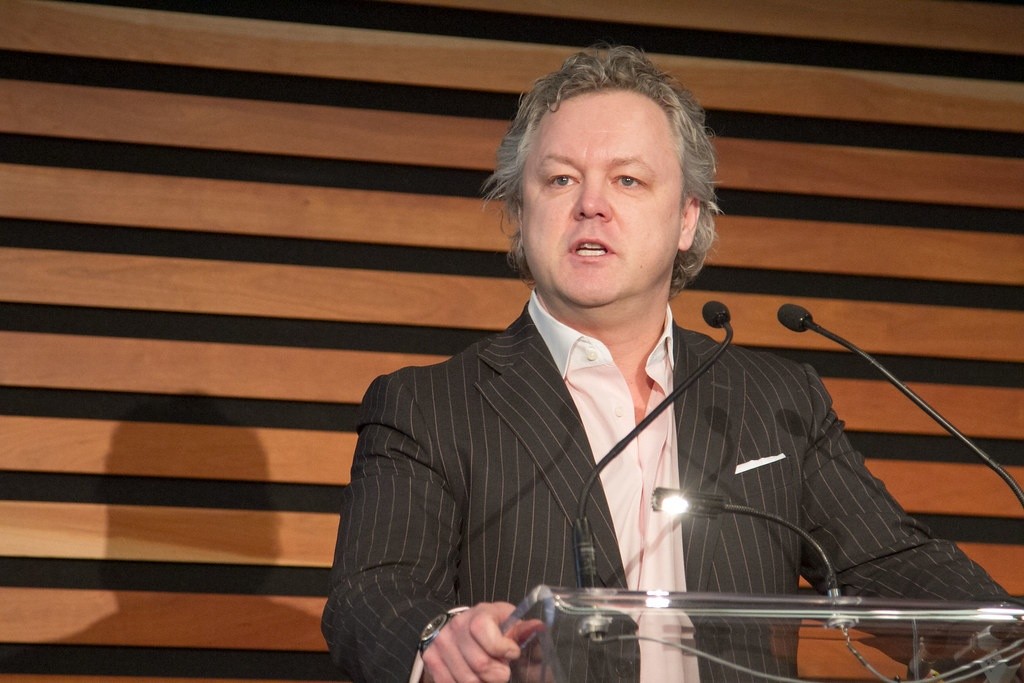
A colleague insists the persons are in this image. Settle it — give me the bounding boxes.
[320,41,1024,683]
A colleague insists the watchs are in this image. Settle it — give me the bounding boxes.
[417,611,457,663]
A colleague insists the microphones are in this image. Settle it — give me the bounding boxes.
[777,303,1024,507]
[571,301,733,588]
